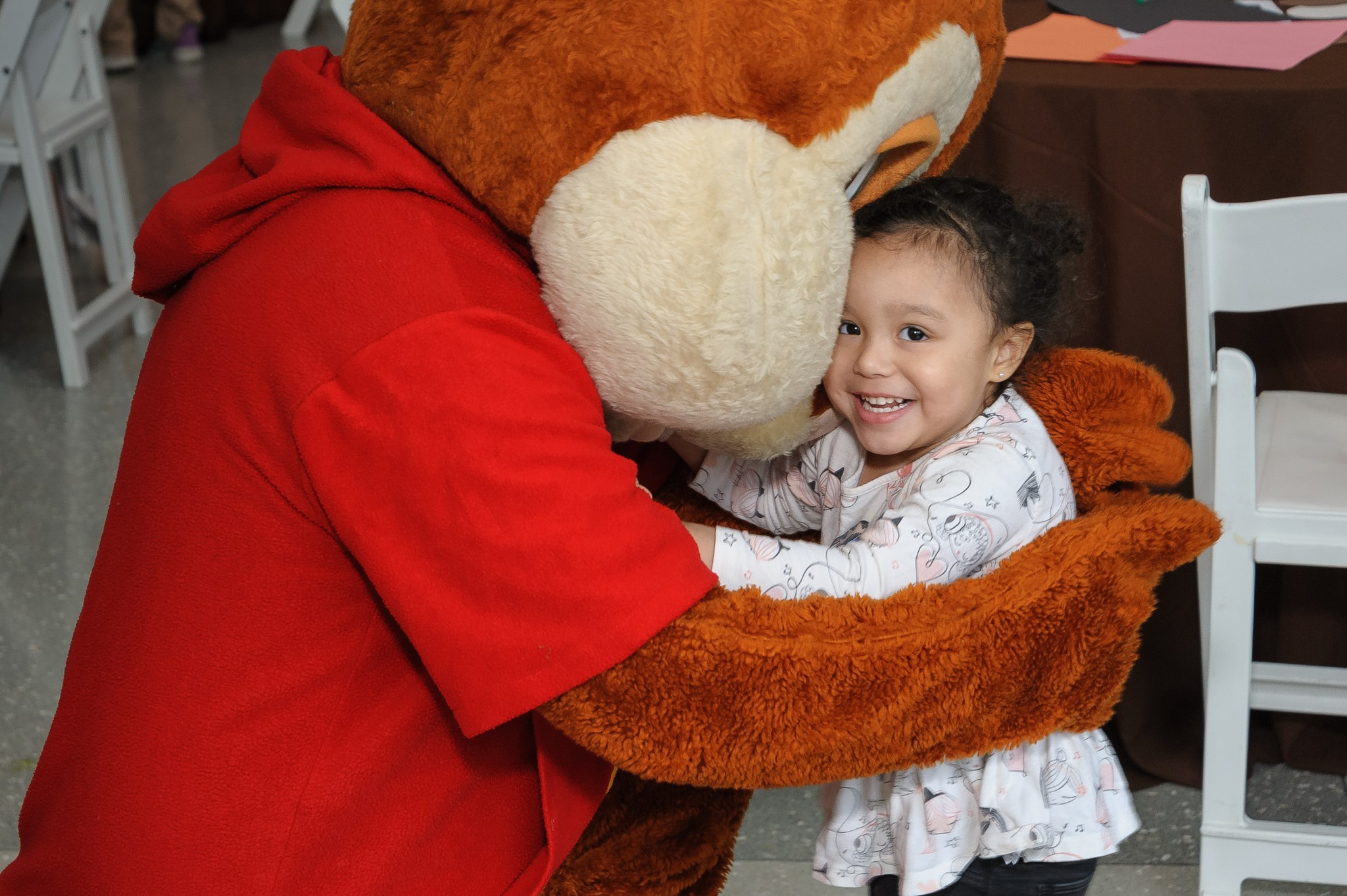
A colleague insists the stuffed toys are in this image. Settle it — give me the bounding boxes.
[0,0,1225,896]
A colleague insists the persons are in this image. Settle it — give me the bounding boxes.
[665,176,1143,896]
[99,0,205,71]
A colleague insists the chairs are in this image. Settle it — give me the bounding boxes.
[0,0,150,390]
[1181,174,1347,896]
